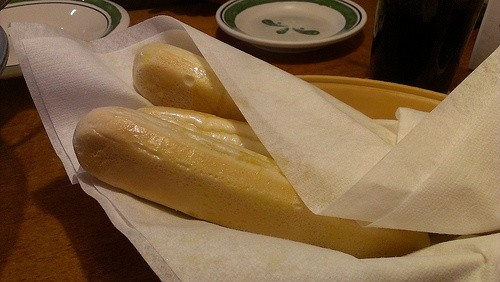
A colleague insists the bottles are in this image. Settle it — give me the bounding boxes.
[366,1,490,98]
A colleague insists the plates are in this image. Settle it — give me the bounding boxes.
[0,1,130,81]
[216,1,368,51]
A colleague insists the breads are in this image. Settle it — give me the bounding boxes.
[73,41,431,261]
